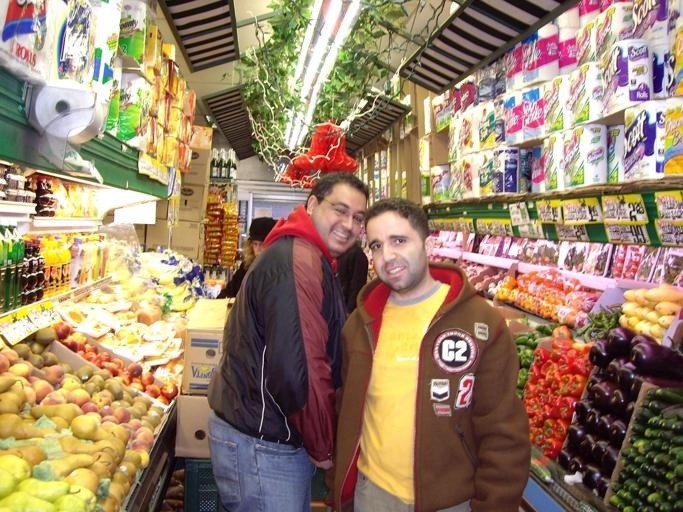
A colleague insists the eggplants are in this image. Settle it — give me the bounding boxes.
[558,329,663,501]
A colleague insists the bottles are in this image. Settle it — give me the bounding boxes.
[211,262,220,280]
[26,175,57,218]
[0,224,109,313]
[208,146,239,180]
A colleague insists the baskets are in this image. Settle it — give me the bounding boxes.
[184,460,221,512]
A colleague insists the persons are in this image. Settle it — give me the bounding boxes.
[330,242,368,313]
[329,197,534,512]
[214,217,279,298]
[205,171,368,512]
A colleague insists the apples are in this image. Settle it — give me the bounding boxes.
[57,323,179,405]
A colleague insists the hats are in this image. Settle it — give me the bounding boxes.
[249,217,278,240]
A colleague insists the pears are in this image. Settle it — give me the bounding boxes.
[0,374,141,511]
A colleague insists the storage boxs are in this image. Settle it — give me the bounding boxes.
[182,125,213,186]
[175,395,212,458]
[156,184,208,221]
[145,220,205,260]
[182,298,236,395]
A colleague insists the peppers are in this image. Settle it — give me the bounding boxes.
[514,326,596,456]
[577,305,620,342]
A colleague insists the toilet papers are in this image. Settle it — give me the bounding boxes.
[418,0,683,205]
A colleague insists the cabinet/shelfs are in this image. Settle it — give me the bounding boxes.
[424,82,682,511]
[0,66,177,512]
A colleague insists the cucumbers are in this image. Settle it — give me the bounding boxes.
[607,388,683,512]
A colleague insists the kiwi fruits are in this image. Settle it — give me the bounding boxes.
[14,326,165,433]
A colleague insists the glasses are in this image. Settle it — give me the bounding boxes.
[321,196,366,227]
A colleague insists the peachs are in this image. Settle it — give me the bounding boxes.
[0,349,155,452]
